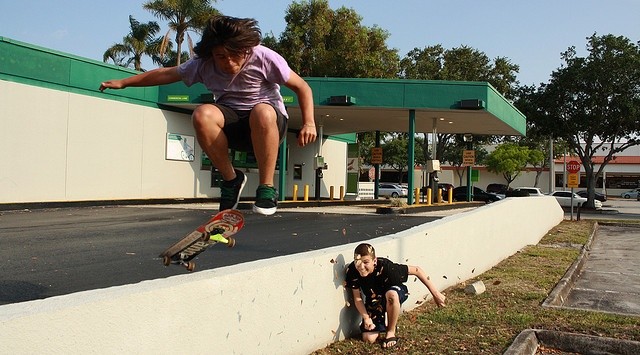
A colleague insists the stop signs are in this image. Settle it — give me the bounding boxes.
[566,161,580,173]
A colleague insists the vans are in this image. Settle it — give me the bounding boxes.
[513,188,543,196]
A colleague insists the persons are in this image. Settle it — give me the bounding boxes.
[99,15,318,216]
[344,243,446,348]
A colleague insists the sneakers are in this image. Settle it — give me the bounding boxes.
[218,169,248,212]
[254,185,279,215]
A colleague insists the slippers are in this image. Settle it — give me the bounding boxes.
[382,336,398,349]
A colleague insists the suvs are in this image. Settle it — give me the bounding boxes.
[434,183,454,201]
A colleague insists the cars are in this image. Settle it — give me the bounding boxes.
[378,184,408,198]
[621,188,640,199]
[576,190,606,202]
[549,191,602,207]
[443,186,502,204]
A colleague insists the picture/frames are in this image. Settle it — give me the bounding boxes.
[165,132,195,162]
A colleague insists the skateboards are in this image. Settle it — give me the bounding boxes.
[158,209,245,271]
